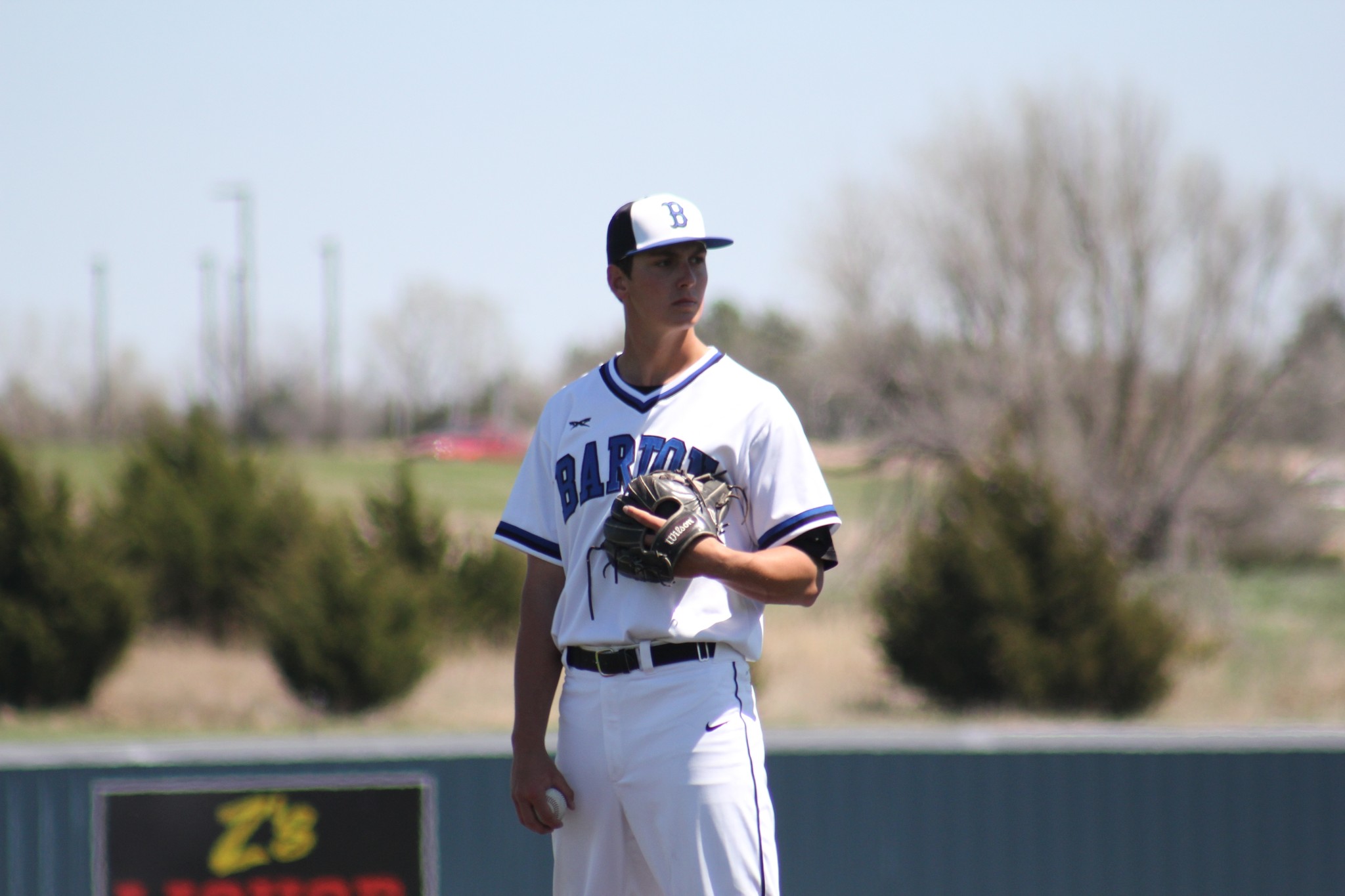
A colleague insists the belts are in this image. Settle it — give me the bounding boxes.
[566,641,718,677]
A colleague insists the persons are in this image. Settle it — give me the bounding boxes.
[496,195,842,896]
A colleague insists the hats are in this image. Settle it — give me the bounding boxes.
[607,192,733,266]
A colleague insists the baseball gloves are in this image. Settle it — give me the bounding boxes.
[601,469,735,583]
